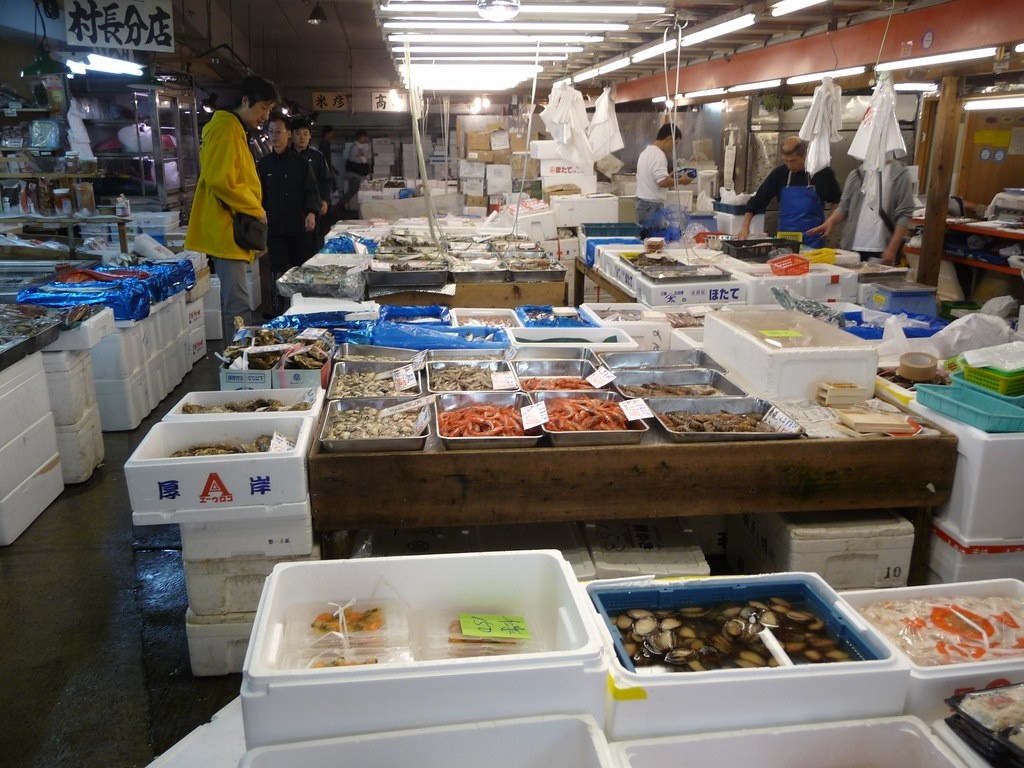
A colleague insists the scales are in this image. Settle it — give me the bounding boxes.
[865,279,940,319]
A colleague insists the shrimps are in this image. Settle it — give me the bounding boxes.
[430,365,776,439]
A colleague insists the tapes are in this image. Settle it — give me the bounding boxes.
[899,351,938,381]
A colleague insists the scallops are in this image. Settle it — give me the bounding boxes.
[325,353,418,439]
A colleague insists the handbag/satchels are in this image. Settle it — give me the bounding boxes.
[233,210,267,251]
[884,239,906,265]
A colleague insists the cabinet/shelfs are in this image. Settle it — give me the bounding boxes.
[71,74,201,212]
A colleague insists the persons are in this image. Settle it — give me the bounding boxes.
[184,74,280,351]
[807,158,915,267]
[737,137,842,249]
[634,123,694,236]
[336,128,368,211]
[255,115,340,319]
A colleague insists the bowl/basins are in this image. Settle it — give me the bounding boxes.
[97,205,116,215]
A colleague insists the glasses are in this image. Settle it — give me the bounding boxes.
[267,129,289,135]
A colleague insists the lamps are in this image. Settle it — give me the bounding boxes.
[552,73,574,88]
[651,94,684,102]
[376,17,631,31]
[390,54,569,61]
[382,32,606,43]
[872,46,998,72]
[678,3,764,47]
[962,97,1024,111]
[307,0,666,26]
[593,52,632,75]
[727,79,784,93]
[399,64,544,91]
[766,0,826,18]
[683,87,728,97]
[572,65,599,83]
[629,33,678,64]
[871,81,941,92]
[386,44,584,53]
[786,65,868,85]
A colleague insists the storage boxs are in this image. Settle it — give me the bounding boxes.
[0,112,1024,768]
